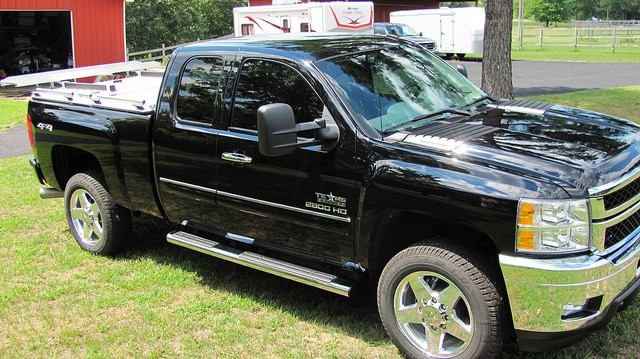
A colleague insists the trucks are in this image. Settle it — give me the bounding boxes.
[388,6,487,57]
[232,2,373,43]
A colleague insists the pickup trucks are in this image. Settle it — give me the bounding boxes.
[25,35,640,359]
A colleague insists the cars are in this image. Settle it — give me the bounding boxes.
[10,44,52,76]
[374,22,436,53]
